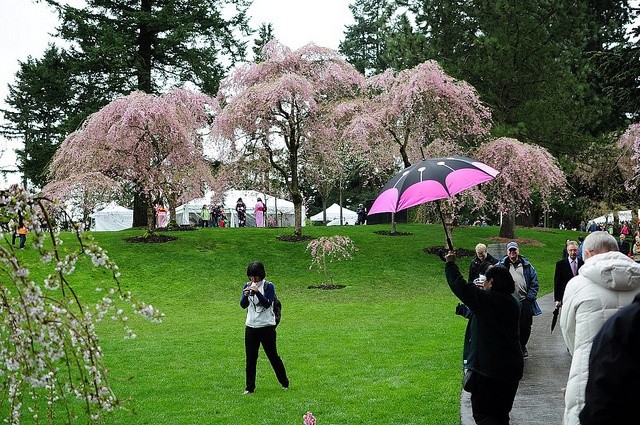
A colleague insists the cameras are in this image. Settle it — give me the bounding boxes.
[249,289,255,296]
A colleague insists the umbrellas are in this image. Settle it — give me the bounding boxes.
[551,302,562,335]
[366,146,501,262]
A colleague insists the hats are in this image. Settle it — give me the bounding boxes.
[507,242,519,249]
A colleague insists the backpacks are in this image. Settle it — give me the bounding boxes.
[263,281,281,325]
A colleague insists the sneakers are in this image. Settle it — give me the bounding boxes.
[523,346,528,358]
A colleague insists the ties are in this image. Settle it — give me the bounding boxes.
[572,261,577,276]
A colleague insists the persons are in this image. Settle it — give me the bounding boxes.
[236,198,246,226]
[632,236,640,263]
[200,205,209,227]
[469,243,498,283]
[156,201,168,227]
[357,204,366,225]
[494,242,542,359]
[579,220,640,234]
[554,241,584,356]
[617,235,630,256]
[240,262,290,395]
[560,231,640,425]
[445,251,524,425]
[211,205,229,228]
[254,199,266,227]
[631,231,640,255]
[7,217,19,245]
[562,239,570,259]
[578,292,640,424]
[17,216,28,250]
[577,236,585,259]
